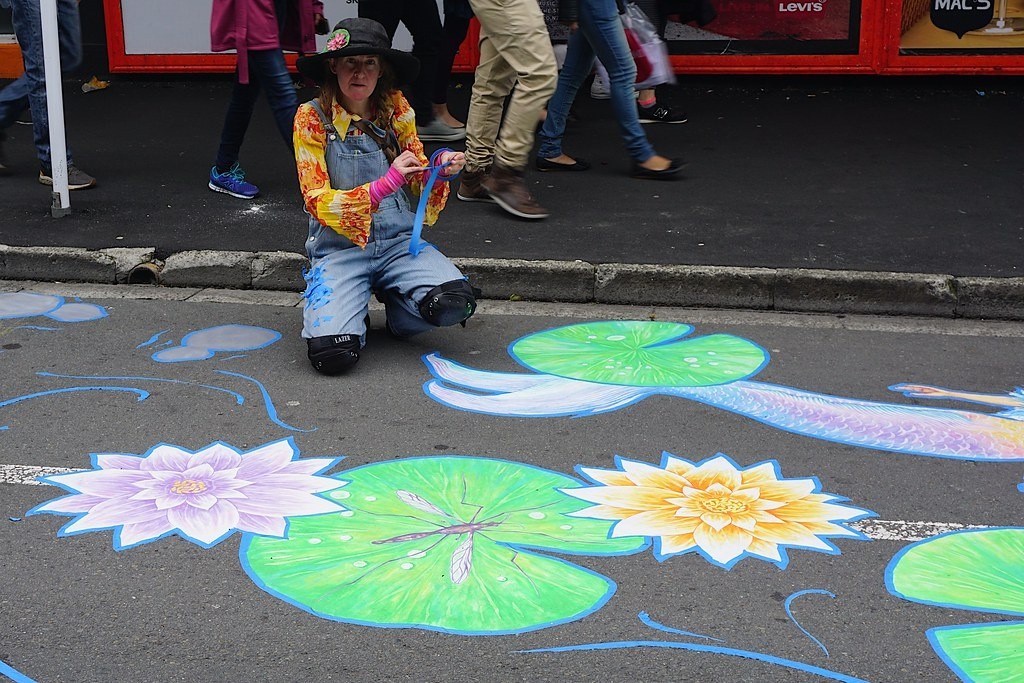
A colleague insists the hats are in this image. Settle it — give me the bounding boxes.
[296,18,421,88]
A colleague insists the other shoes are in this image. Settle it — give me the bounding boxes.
[435,116,466,130]
[631,156,695,178]
[416,118,467,141]
[590,72,639,99]
[536,152,591,171]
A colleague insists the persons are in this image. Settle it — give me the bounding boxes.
[457,0,559,218]
[433,0,474,128]
[358,0,466,142]
[591,0,688,123]
[0,0,95,189]
[536,0,690,178]
[293,17,476,375]
[208,0,330,199]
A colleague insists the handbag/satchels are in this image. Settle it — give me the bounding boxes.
[618,14,654,84]
[596,4,671,93]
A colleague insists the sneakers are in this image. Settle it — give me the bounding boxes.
[208,161,260,199]
[486,166,550,218]
[0,161,7,168]
[636,103,688,123]
[38,163,96,189]
[456,170,497,203]
